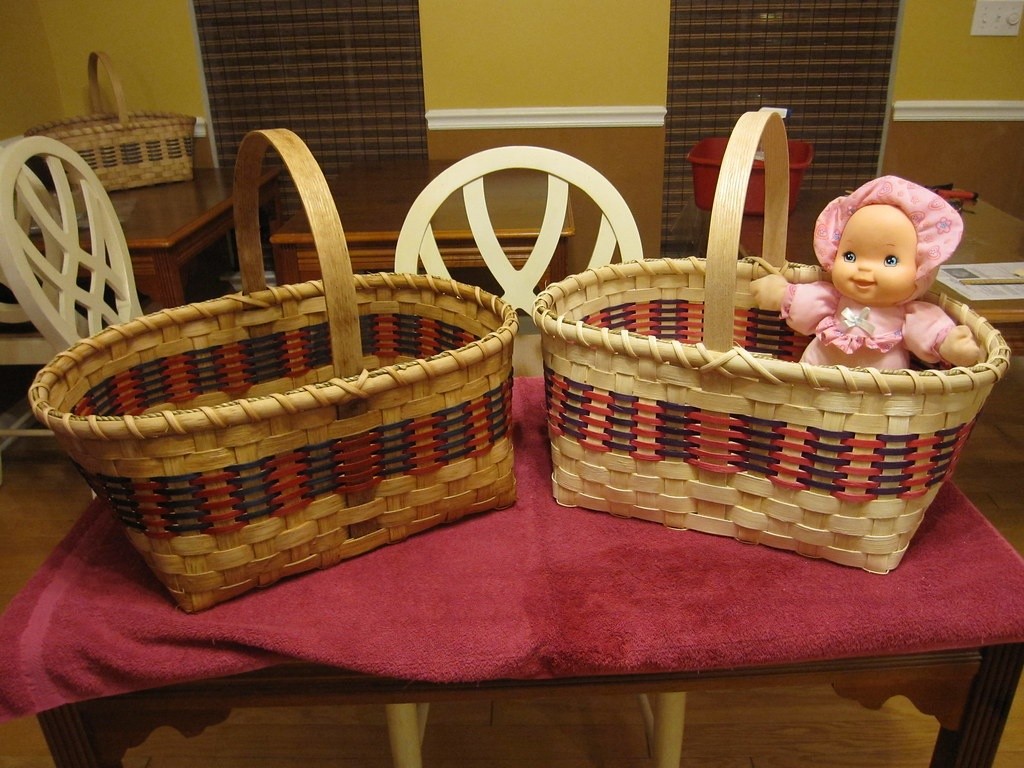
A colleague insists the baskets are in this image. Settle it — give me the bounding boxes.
[532,110,1010,573]
[24,49,196,191]
[28,129,519,615]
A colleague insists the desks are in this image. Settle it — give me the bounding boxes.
[753,191,1024,358]
[0,376,1024,768]
[266,157,577,288]
[35,167,284,310]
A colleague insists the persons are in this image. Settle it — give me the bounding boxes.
[749,175,980,371]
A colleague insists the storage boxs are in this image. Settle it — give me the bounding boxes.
[685,136,818,215]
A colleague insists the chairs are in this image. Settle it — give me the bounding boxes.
[383,146,689,768]
[0,136,145,355]
